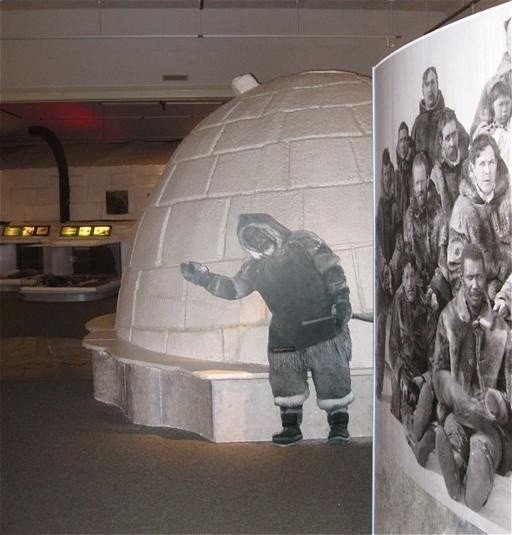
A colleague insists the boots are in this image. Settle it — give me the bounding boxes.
[273,408,302,446]
[327,409,350,443]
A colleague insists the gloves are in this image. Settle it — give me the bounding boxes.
[338,299,353,327]
[180,261,209,284]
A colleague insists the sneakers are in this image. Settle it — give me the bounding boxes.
[403,428,493,512]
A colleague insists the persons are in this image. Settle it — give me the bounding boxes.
[180,209,354,446]
[376,19,512,511]
